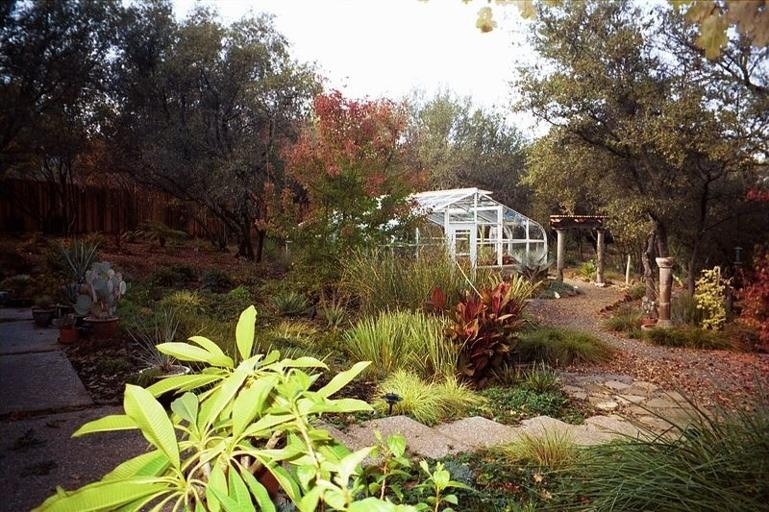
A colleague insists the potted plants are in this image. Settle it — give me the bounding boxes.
[126,308,206,405]
[52,317,79,344]
[31,294,55,327]
[73,260,126,340]
[54,241,102,319]
